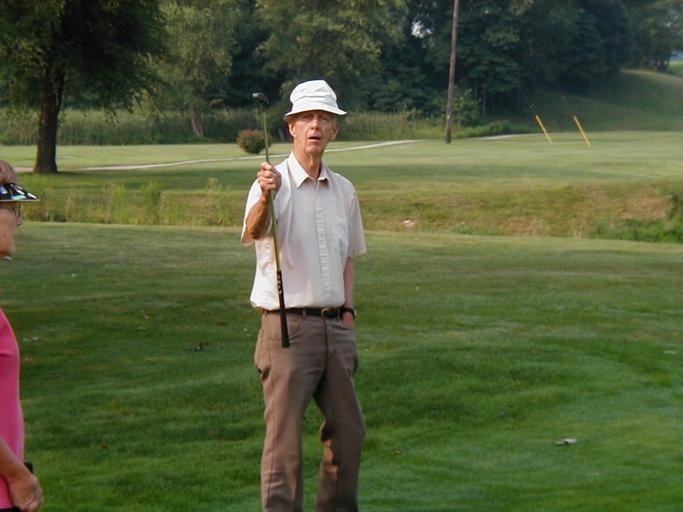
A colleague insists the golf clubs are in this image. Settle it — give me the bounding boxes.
[252,92,290,348]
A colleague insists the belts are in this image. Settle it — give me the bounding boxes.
[266,306,342,320]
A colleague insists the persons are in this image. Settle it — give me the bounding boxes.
[239,76,369,511]
[0,156,48,512]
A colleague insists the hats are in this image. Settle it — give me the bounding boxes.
[283,78,348,119]
[0,182,41,203]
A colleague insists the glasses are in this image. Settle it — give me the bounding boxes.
[2,202,22,218]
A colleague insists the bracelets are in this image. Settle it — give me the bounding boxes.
[343,306,356,319]
